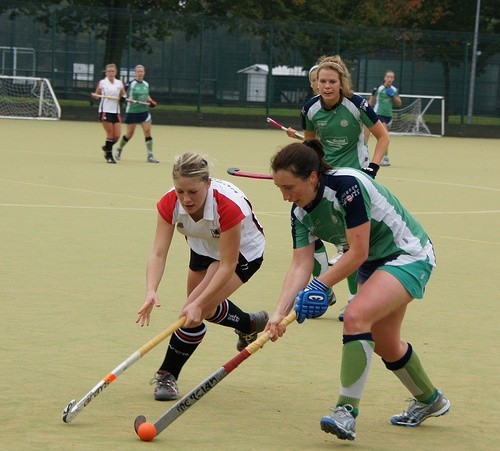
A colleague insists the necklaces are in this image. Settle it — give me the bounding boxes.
[326,100,339,114]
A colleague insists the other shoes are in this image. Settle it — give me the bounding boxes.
[381,155,390,166]
[147,155,160,163]
[115,144,122,160]
[328,290,336,306]
[102,146,106,151]
[338,313,344,322]
[104,153,116,164]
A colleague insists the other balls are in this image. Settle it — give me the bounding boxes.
[138,421,157,442]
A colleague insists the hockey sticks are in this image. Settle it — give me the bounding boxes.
[62,316,187,423]
[227,168,274,180]
[91,91,158,106]
[267,117,305,140]
[133,312,297,437]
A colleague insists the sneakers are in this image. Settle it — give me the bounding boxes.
[320,404,357,441]
[391,388,450,427]
[150,370,179,400]
[234,311,269,352]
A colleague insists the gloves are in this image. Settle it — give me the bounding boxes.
[361,162,380,179]
[150,101,157,107]
[386,87,396,97]
[119,97,125,102]
[295,276,329,324]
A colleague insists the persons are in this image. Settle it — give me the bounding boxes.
[370,70,402,166]
[302,53,390,323]
[308,63,370,173]
[91,63,127,164]
[135,153,270,401]
[257,139,451,441]
[114,64,160,162]
